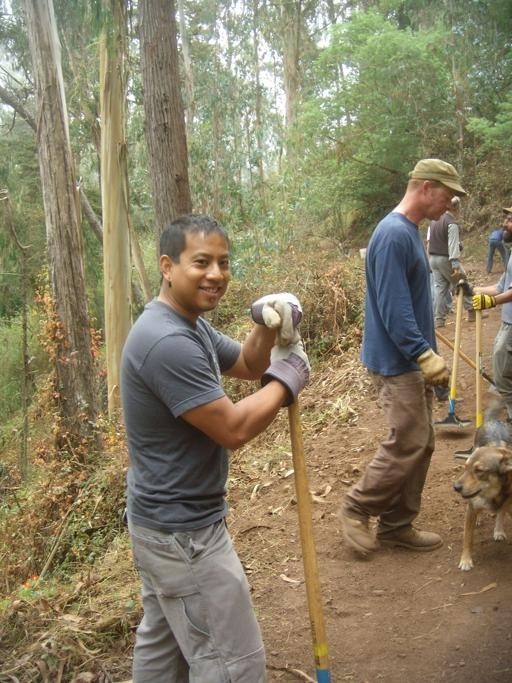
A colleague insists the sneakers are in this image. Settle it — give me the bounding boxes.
[376,525,444,551]
[466,307,490,323]
[337,504,379,555]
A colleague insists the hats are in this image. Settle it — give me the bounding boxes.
[407,158,467,198]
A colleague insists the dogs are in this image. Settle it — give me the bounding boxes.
[434,418,512,572]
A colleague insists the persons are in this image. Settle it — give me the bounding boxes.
[121,214,310,683]
[459,205,512,427]
[425,195,489,324]
[338,159,467,558]
[484,227,507,273]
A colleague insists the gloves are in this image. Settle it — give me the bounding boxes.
[472,293,496,310]
[260,340,312,409]
[416,347,450,388]
[251,291,304,348]
[455,278,473,296]
